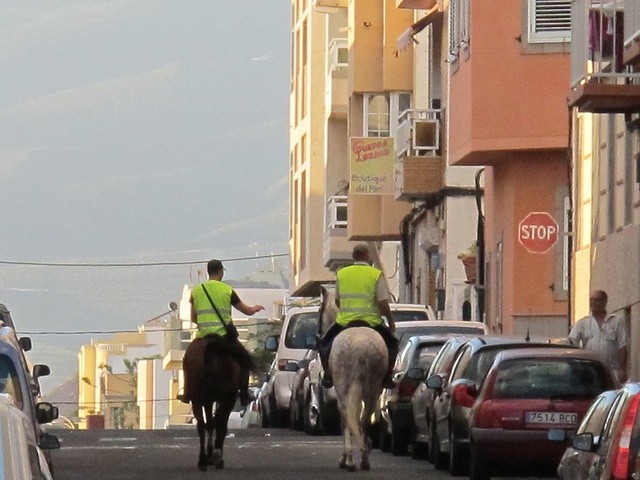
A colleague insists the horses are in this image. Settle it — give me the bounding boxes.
[183,334,242,471]
[313,284,390,470]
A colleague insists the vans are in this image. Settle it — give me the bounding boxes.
[274,306,321,429]
[388,303,436,322]
[394,321,487,350]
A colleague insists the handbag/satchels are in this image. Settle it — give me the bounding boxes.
[225,324,240,339]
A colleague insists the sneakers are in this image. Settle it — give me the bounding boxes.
[176,394,187,403]
[241,396,256,404]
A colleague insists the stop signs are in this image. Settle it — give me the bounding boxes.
[518,212,559,254]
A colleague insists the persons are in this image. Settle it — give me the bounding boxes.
[570,290,628,387]
[177,260,264,405]
[316,243,399,390]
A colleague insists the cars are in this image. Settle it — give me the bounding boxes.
[0,303,75,480]
[303,352,340,435]
[227,358,277,428]
[433,338,581,476]
[557,381,640,480]
[288,349,318,431]
[411,336,474,464]
[468,349,620,480]
[380,336,450,456]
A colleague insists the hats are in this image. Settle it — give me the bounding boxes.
[208,259,226,272]
[353,244,371,256]
